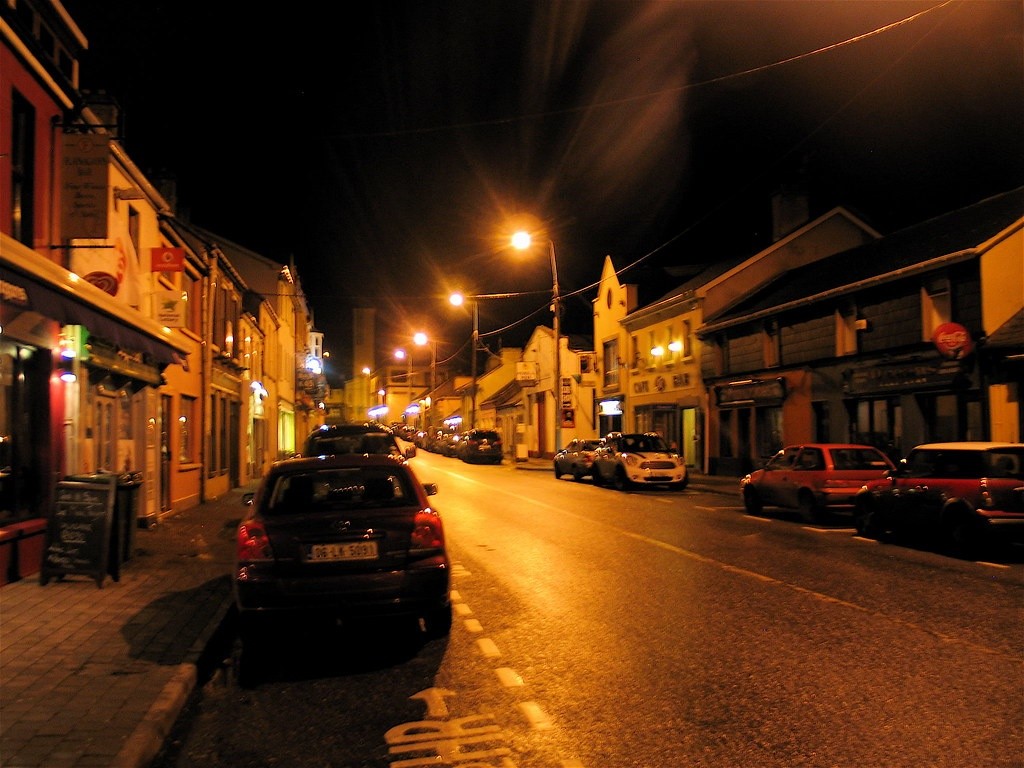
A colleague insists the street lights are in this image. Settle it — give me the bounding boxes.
[450,295,476,429]
[416,333,436,428]
[510,232,565,457]
[393,350,413,406]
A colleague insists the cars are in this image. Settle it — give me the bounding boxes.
[854,440,1024,557]
[552,438,599,484]
[741,444,897,524]
[234,452,454,638]
[390,422,503,465]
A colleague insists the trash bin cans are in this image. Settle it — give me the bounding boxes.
[63,469,144,566]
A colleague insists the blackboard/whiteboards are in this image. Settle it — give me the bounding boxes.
[41,482,114,577]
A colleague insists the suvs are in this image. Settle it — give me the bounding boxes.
[301,421,419,460]
[590,430,690,492]
